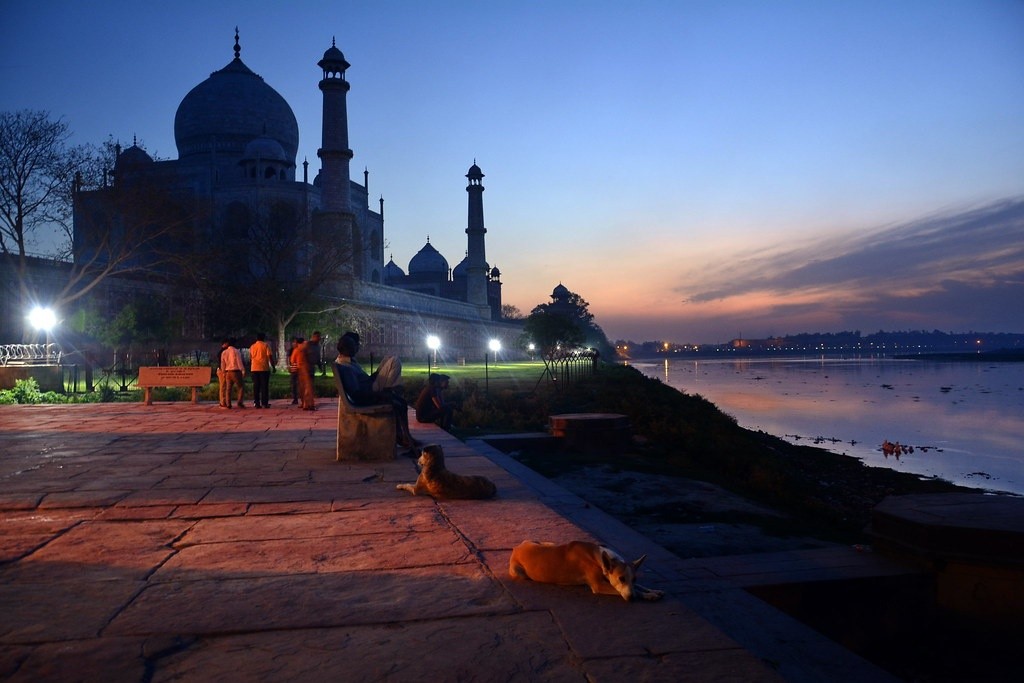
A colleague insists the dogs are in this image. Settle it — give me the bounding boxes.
[508,540,665,604]
[397,445,497,500]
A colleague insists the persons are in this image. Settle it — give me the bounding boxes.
[216,336,246,409]
[335,331,425,447]
[248,333,277,408]
[416,374,457,429]
[286,331,322,410]
[442,363,1024,683]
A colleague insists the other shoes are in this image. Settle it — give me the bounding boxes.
[219,404,226,409]
[302,405,317,411]
[262,403,272,408]
[227,404,232,409]
[292,399,299,405]
[237,402,245,409]
[399,436,422,447]
[253,402,262,408]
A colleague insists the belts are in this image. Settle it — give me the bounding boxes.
[226,369,239,373]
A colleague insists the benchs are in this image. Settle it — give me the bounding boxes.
[133,366,212,405]
[331,357,397,464]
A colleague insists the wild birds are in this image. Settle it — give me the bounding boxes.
[882,440,928,454]
